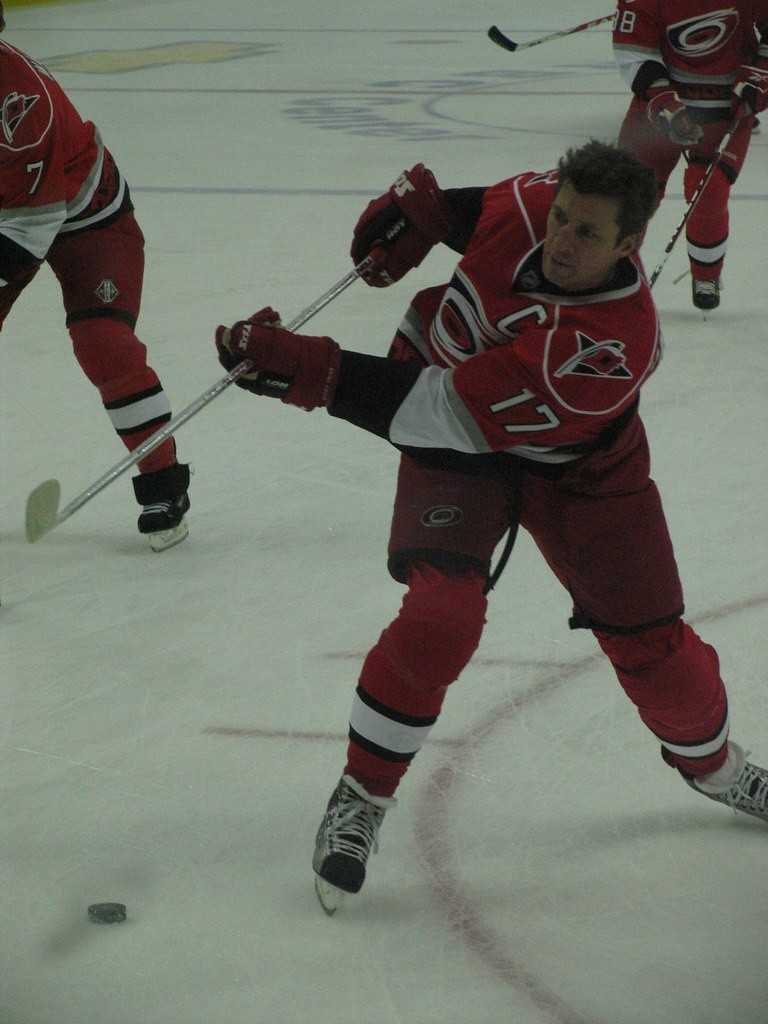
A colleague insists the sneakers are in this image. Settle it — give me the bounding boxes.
[692,277,720,321]
[132,438,190,553]
[312,774,397,915]
[677,739,768,823]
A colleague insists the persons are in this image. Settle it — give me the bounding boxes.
[611,0,768,309]
[213,141,767,918]
[0,0,190,553]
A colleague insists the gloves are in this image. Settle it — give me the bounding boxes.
[351,164,459,288]
[731,53,768,120]
[215,306,340,412]
[645,83,704,156]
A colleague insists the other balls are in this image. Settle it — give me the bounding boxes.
[88,902,127,922]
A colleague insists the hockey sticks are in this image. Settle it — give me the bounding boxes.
[648,111,747,293]
[487,14,616,53]
[23,239,390,545]
